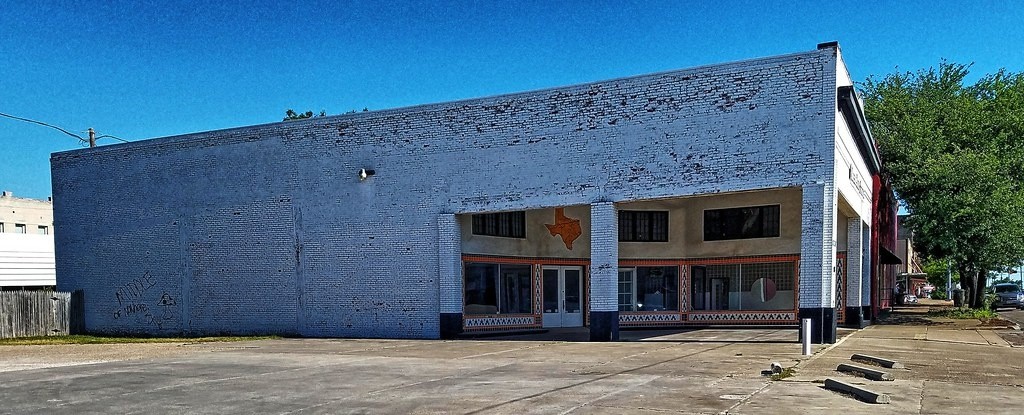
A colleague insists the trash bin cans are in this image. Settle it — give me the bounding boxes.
[952,289,966,307]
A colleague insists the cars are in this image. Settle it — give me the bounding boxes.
[988,283,1024,310]
